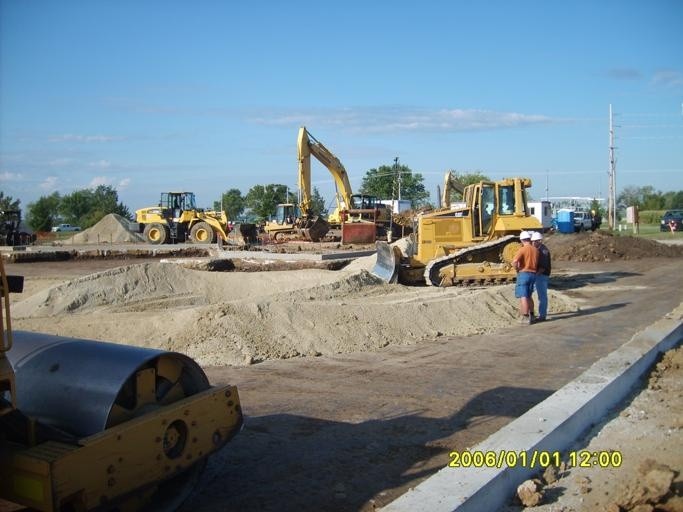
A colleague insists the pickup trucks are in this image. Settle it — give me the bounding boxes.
[51,223,81,232]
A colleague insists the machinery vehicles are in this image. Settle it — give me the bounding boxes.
[128,191,258,247]
[263,125,488,242]
[1,254,243,512]
[368,177,545,288]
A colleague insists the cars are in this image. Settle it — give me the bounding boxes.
[573,211,592,231]
[660,210,683,232]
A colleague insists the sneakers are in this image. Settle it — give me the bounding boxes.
[517,316,534,323]
[534,316,546,322]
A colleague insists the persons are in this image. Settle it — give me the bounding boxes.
[512,230,539,325]
[528,231,551,321]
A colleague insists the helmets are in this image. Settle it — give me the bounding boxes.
[530,232,543,241]
[520,231,531,239]
